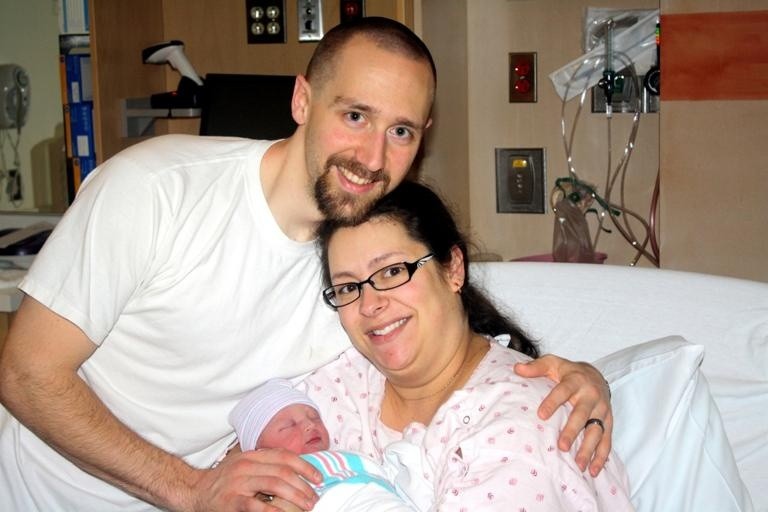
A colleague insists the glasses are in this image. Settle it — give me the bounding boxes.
[323,252,438,309]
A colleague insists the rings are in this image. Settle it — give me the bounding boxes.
[585,418,604,434]
[254,491,276,503]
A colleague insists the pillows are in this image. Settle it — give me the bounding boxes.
[592,336,752,512]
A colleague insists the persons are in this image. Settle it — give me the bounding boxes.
[227,378,420,512]
[299,176,635,512]
[0,15,614,512]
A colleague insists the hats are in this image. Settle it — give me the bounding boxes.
[228,378,320,452]
